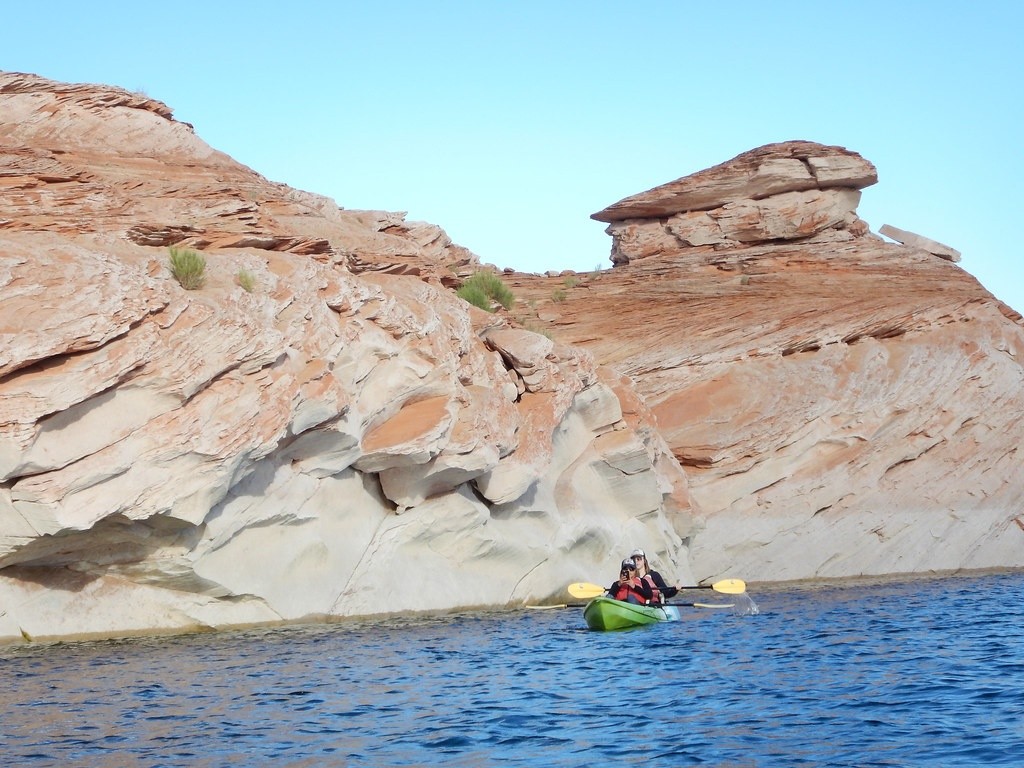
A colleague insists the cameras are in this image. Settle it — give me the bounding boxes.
[623,570,630,580]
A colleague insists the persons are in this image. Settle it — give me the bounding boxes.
[606,558,653,606]
[630,549,682,604]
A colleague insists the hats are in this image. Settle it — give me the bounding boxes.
[630,549,644,558]
[622,559,636,568]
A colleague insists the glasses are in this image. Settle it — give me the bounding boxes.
[622,566,635,571]
[634,557,642,561]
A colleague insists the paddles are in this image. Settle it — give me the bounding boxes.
[525,602,737,611]
[566,578,746,600]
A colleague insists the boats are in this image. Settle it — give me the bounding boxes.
[583,598,681,630]
